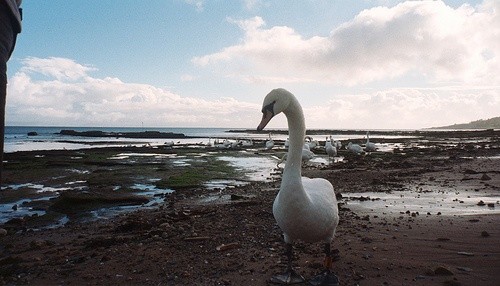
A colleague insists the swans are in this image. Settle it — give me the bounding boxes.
[166,131,365,168]
[257,88,339,284]
[365,131,376,152]
